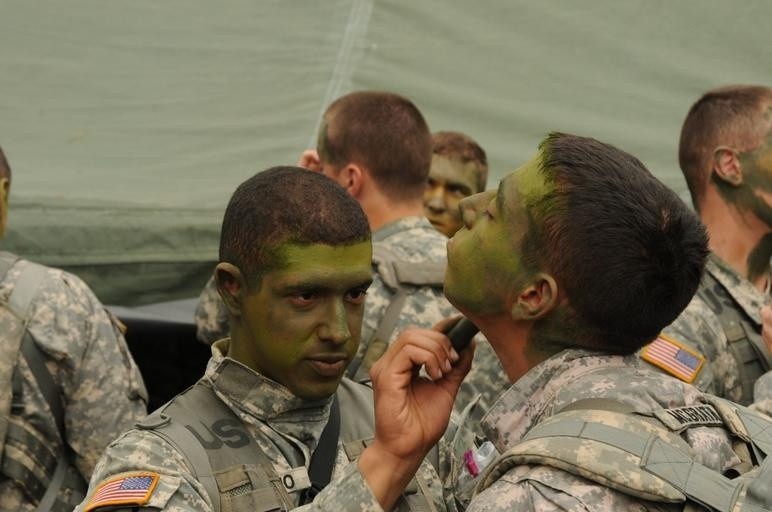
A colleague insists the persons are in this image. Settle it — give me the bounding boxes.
[423,132,490,237]
[442,128,771,512]
[633,81,772,407]
[74,164,478,512]
[0,146,149,512]
[194,90,510,422]
